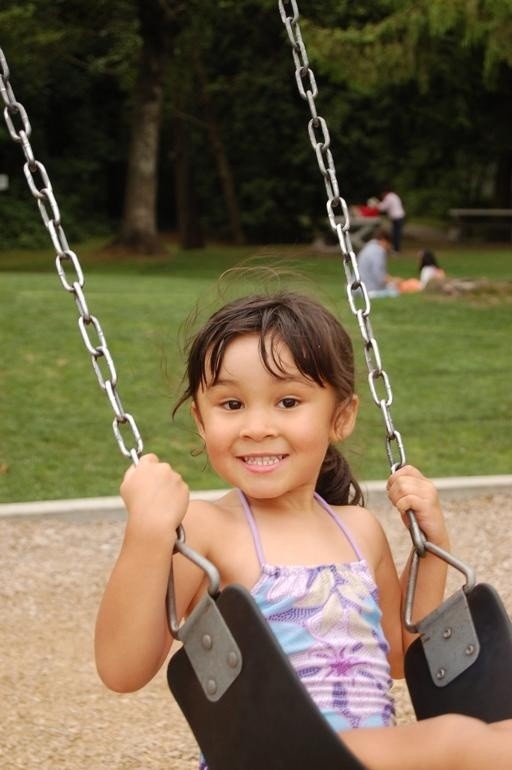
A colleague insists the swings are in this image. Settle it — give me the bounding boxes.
[1,2,511,765]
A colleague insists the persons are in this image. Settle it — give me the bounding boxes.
[360,234,398,292]
[374,187,406,250]
[91,293,512,769]
[400,248,443,293]
